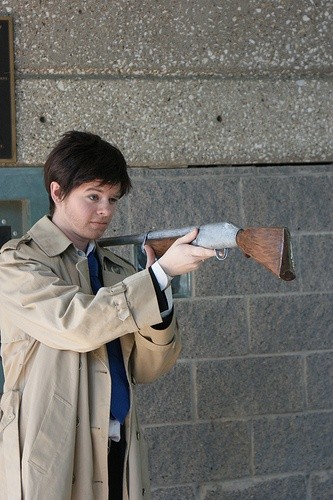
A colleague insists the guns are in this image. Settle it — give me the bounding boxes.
[96,219,298,283]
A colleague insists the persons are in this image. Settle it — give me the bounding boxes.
[1,130,227,500]
